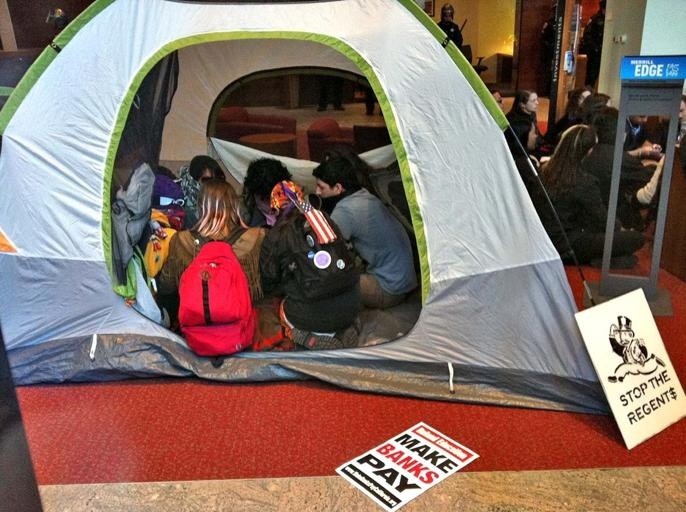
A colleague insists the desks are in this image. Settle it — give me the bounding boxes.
[237,133,298,159]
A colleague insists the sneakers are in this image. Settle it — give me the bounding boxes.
[292,324,358,350]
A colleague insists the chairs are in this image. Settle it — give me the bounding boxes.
[215,109,297,144]
[307,118,354,163]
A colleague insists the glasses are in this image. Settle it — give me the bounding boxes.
[153,196,185,208]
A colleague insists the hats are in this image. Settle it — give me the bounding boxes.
[153,176,184,196]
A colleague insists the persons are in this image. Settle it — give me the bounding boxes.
[438,1,462,44]
[582,1,608,85]
[122,154,417,355]
[363,87,385,117]
[488,85,686,268]
[42,5,67,40]
[315,75,348,112]
[539,3,558,94]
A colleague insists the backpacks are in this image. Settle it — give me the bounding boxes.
[177,227,259,357]
[261,210,358,304]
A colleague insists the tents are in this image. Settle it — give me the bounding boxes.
[0,0,609,418]
